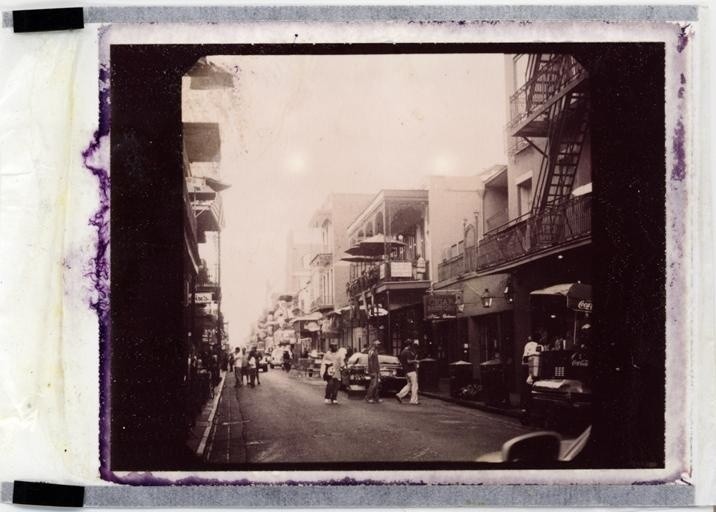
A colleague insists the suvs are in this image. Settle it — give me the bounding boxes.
[246,349,269,372]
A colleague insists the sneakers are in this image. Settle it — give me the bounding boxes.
[365,395,383,403]
[395,394,402,402]
[410,402,420,405]
[324,399,338,404]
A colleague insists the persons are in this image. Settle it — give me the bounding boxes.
[395,338,422,405]
[189,339,384,405]
[519,331,540,413]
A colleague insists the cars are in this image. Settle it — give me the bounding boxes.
[340,351,407,393]
[269,348,294,369]
